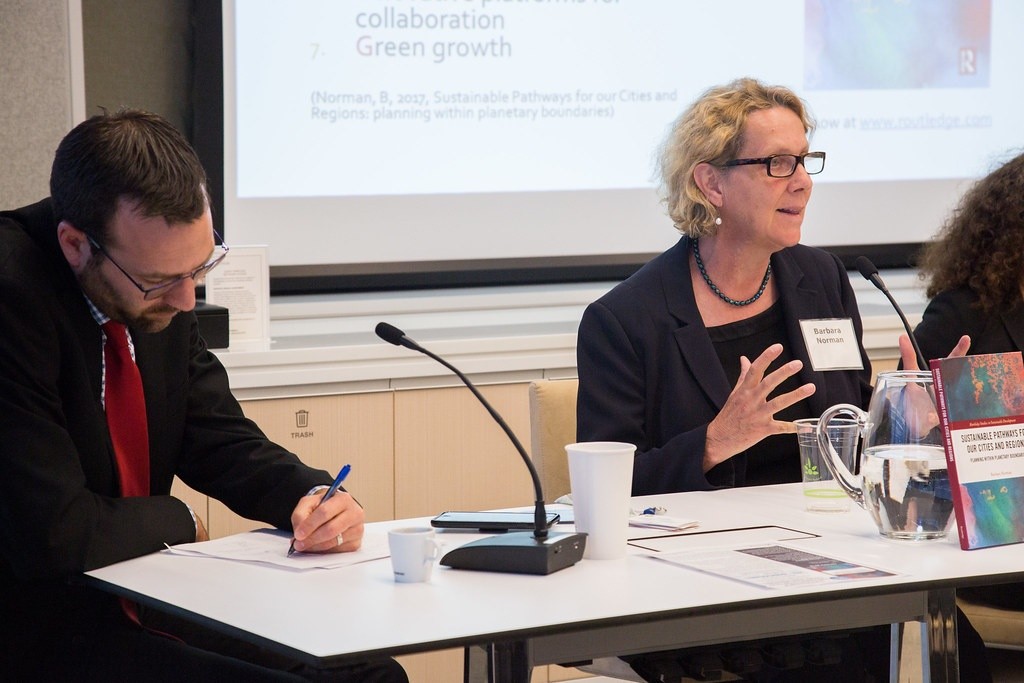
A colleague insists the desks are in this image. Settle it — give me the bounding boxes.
[921,599,1024,683]
[78,479,1024,683]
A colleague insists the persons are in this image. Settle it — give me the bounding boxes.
[577,80,971,683]
[0,105,409,683]
[897,152,1024,613]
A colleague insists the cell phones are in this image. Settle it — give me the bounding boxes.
[430,508,562,530]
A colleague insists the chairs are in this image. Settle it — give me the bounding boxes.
[528,379,580,507]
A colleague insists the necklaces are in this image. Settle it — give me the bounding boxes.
[694,236,771,306]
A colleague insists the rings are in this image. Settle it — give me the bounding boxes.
[336,534,343,546]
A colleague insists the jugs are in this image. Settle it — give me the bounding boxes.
[817,370,956,541]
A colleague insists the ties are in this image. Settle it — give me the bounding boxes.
[101,322,187,645]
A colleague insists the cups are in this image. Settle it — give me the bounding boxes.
[564,441,637,558]
[388,527,440,584]
[794,418,859,513]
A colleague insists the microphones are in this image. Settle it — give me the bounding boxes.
[373,322,586,577]
[855,255,929,370]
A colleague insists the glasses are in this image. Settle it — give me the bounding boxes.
[86,229,230,301]
[712,150,826,180]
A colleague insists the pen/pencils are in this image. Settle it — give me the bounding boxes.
[286,464,352,557]
